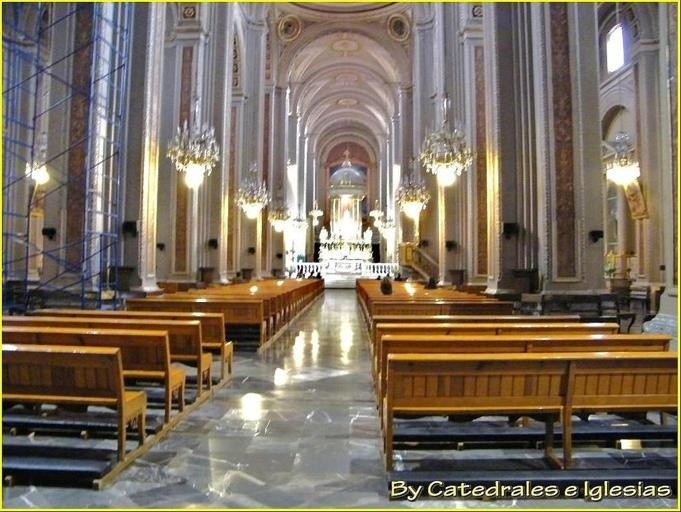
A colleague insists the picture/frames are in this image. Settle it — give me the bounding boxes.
[623,180,650,220]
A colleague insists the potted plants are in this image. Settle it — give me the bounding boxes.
[509,228,539,293]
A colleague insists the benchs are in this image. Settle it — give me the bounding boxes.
[356,277,678,498]
[3,277,325,491]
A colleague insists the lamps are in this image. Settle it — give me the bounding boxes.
[25,162,50,185]
[396,3,430,219]
[370,199,384,227]
[603,77,641,186]
[234,2,324,233]
[166,2,221,190]
[417,3,474,186]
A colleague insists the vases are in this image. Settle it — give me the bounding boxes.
[449,269,464,286]
[198,267,214,284]
[273,268,281,278]
[110,266,134,290]
[241,268,254,281]
